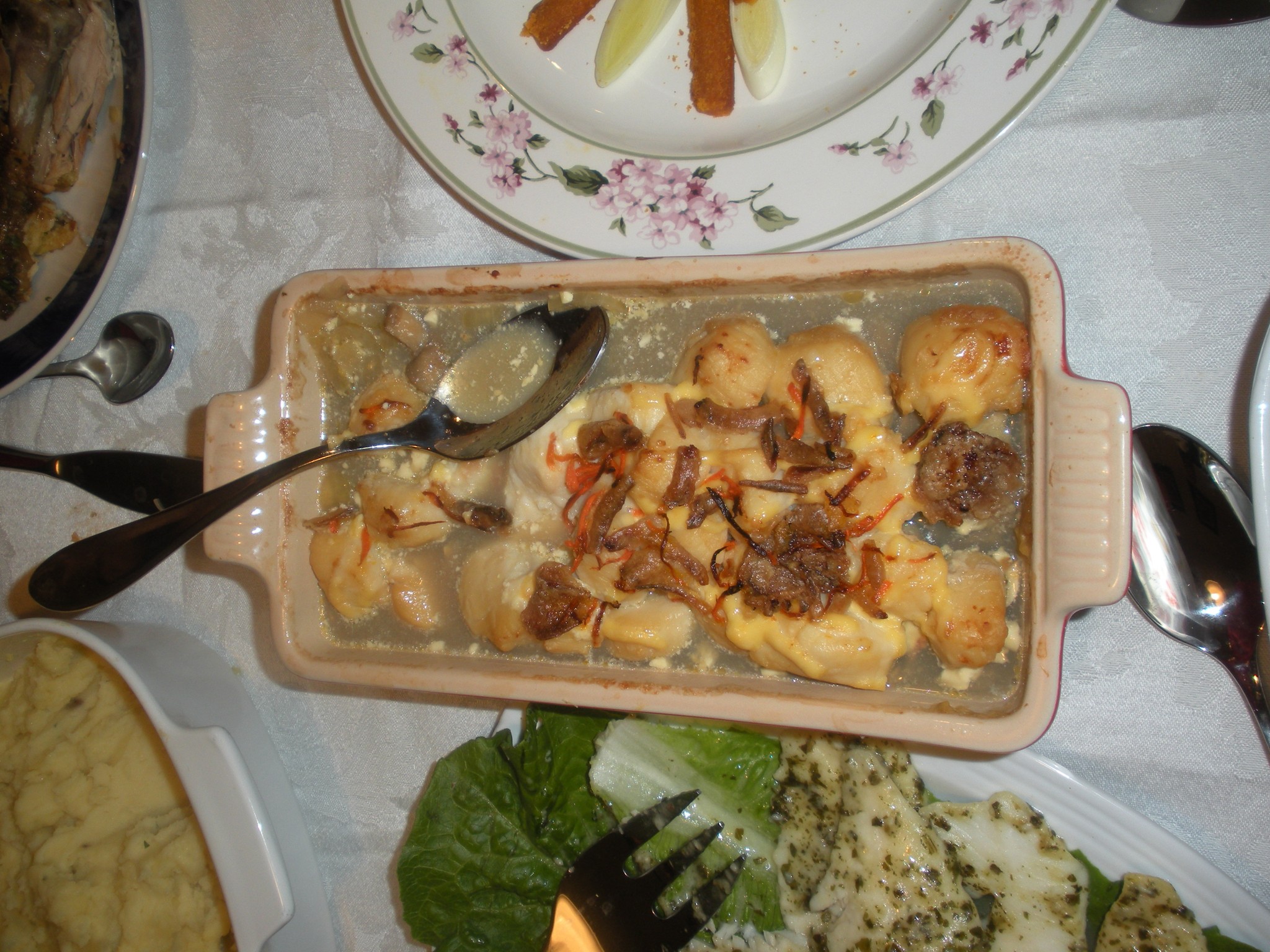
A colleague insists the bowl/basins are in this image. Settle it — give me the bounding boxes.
[1,617,337,952]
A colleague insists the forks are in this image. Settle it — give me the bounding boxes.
[547,789,746,952]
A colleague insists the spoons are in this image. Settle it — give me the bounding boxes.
[32,312,175,405]
[1128,424,1270,762]
[0,443,204,516]
[27,302,610,613]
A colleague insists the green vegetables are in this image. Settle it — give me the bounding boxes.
[393,702,790,951]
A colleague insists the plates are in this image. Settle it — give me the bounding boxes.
[488,694,1270,952]
[0,0,152,400]
[330,0,1118,260]
[204,236,1131,756]
